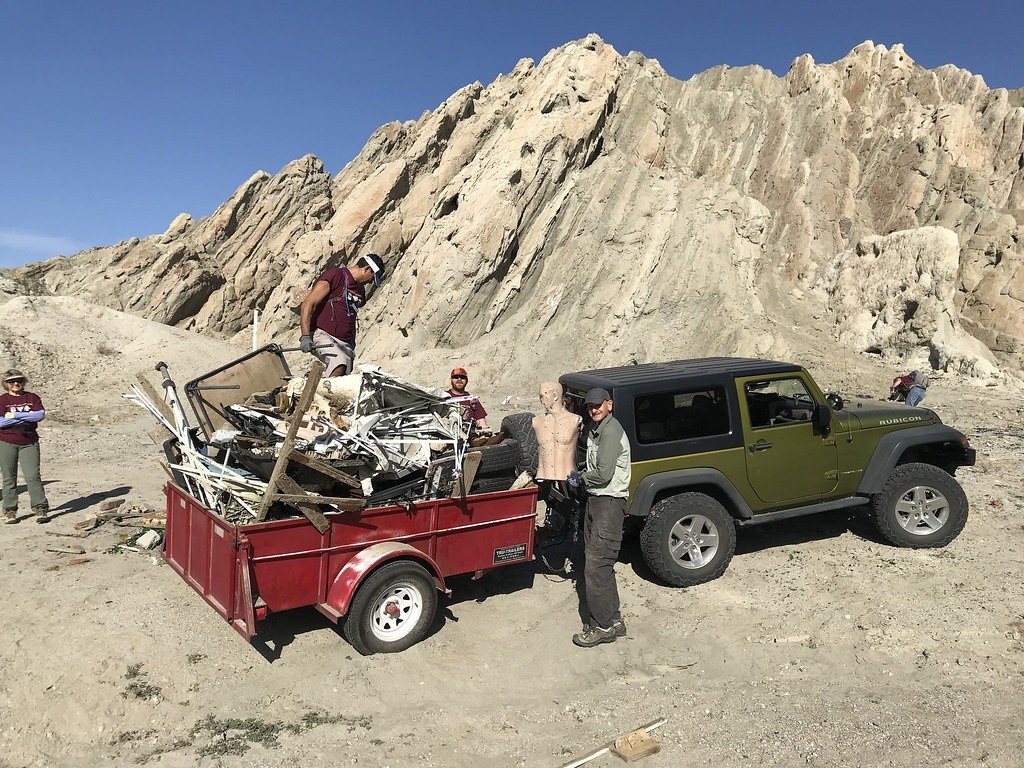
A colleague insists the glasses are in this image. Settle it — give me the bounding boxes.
[4,378,26,384]
[452,376,466,379]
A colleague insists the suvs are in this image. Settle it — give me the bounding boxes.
[552,354,978,589]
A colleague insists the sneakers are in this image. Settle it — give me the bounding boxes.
[583,619,626,636]
[572,626,617,647]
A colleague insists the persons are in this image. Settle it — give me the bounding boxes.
[444,367,490,431]
[300,253,385,378]
[889,376,925,407]
[0,368,50,524]
[566,386,632,647]
[532,380,583,481]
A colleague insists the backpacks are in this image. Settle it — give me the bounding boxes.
[907,369,931,388]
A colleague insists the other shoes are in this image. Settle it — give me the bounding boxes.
[4,508,17,523]
[36,508,49,522]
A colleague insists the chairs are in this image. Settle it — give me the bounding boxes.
[746,397,772,427]
[721,396,734,431]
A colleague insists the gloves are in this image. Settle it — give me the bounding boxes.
[890,387,896,394]
[568,473,583,488]
[299,335,316,353]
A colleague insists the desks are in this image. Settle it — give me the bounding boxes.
[184,342,335,441]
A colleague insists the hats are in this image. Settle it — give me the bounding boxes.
[2,369,27,384]
[582,387,610,406]
[451,368,467,377]
[363,255,384,287]
